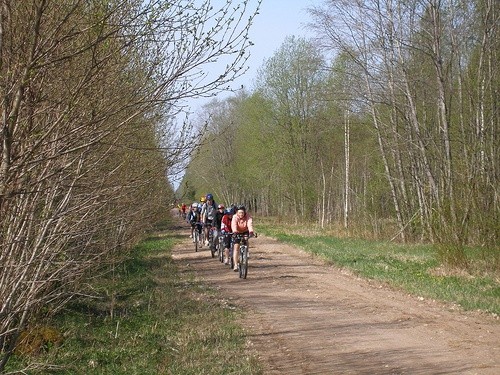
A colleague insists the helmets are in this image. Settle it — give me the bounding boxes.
[235,204,245,211]
[225,208,234,214]
[218,204,226,209]
[193,203,198,207]
[206,194,212,200]
[201,197,207,201]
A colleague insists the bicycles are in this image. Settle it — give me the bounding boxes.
[192,221,257,279]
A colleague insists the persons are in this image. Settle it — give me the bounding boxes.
[177,194,240,264]
[231,205,253,271]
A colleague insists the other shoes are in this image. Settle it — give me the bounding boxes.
[189,235,193,238]
[233,266,238,270]
[199,243,202,247]
[212,246,217,252]
[205,241,210,246]
[225,259,229,264]
